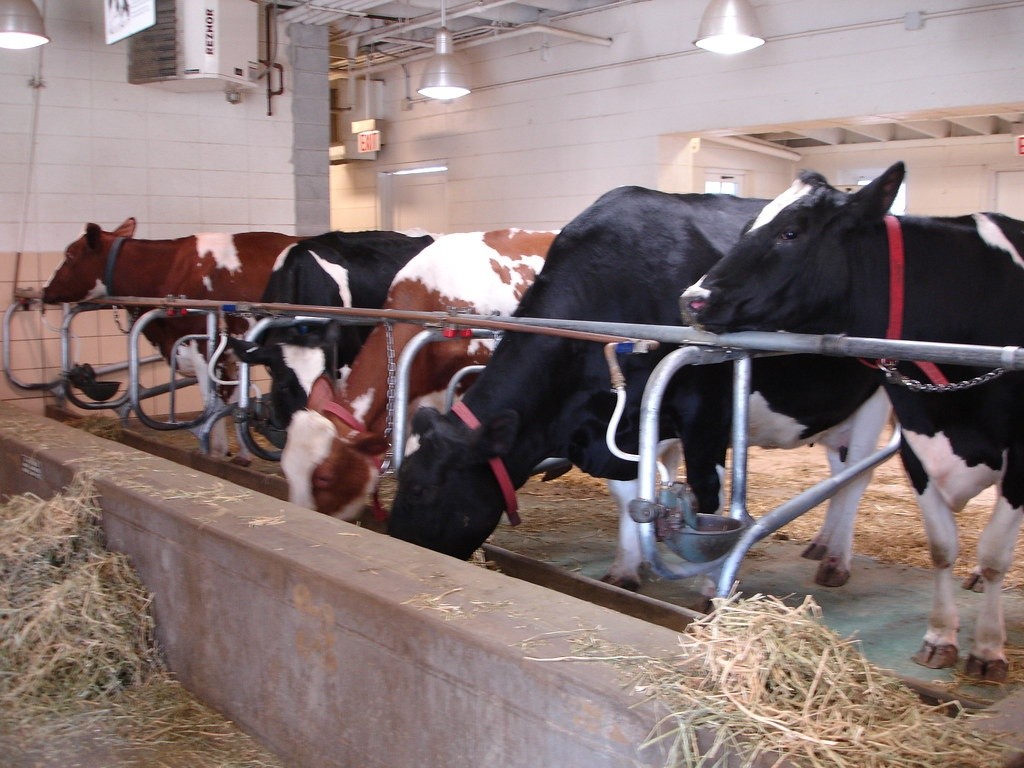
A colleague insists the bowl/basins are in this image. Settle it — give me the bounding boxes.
[660,513,745,563]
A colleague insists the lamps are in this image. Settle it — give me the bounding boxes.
[416,0,474,100]
[0,0,51,50]
[692,0,766,57]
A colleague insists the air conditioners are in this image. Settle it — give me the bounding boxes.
[127,0,262,92]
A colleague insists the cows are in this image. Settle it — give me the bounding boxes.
[41,162,1024,682]
